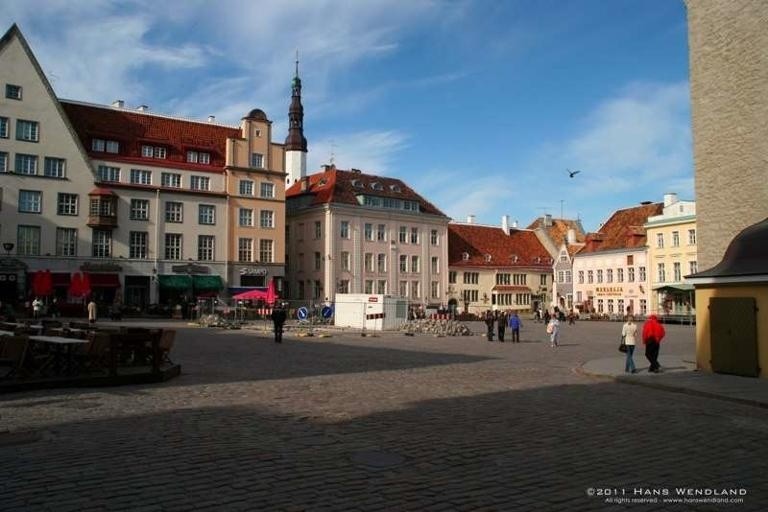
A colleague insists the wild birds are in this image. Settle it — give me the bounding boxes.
[565,169,581,178]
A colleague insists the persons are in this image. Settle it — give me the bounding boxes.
[87,300,98,324]
[509,312,524,343]
[642,315,665,373]
[546,313,562,348]
[621,315,638,374]
[271,303,287,344]
[31,296,44,317]
[483,312,496,341]
[533,307,549,323]
[107,298,122,321]
[496,312,508,342]
[567,309,576,328]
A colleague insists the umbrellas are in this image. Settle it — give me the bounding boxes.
[266,280,276,304]
[33,269,92,304]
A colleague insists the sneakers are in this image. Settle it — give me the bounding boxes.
[625,364,662,373]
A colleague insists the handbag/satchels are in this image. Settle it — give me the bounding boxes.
[547,324,553,333]
[619,336,628,352]
[647,336,656,350]
